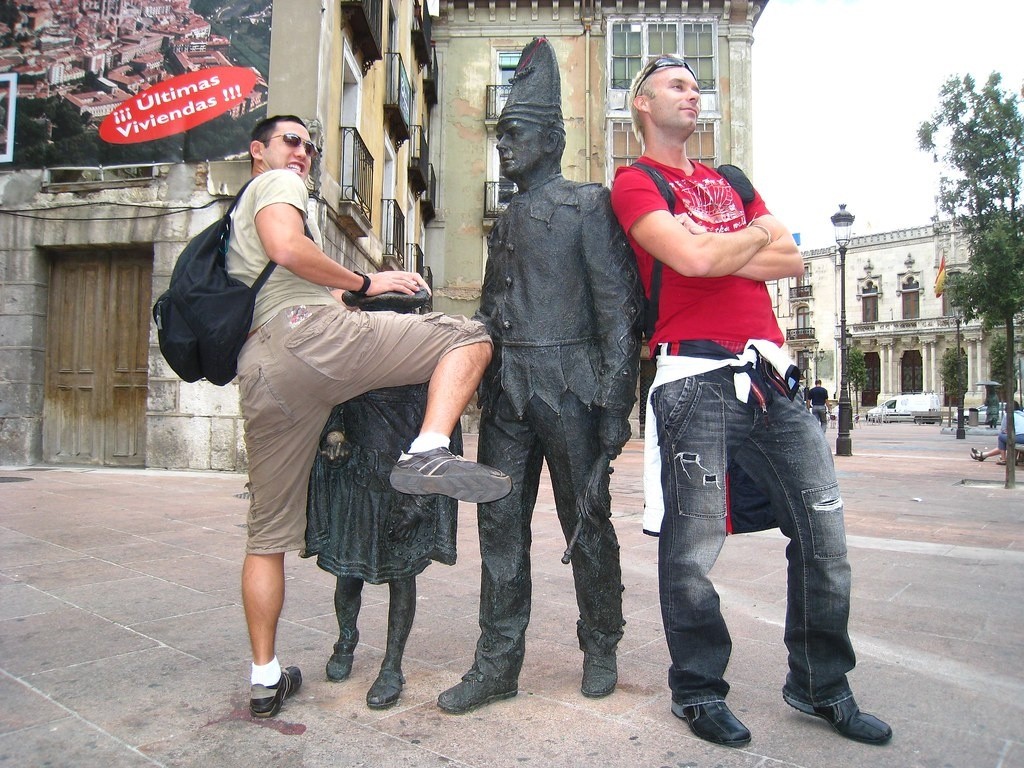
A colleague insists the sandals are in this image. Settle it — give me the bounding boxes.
[970,447,985,461]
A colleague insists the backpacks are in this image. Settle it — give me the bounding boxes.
[152,179,278,389]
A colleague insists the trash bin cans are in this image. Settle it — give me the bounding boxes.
[968,408,978,426]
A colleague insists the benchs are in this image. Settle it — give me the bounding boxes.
[911,411,943,425]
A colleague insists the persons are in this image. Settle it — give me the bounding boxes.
[806,380,832,432]
[437,39,645,712]
[226,115,512,717]
[610,55,892,747]
[984,386,999,428]
[970,400,1024,465]
[298,288,463,709]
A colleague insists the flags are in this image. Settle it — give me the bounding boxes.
[934,255,946,298]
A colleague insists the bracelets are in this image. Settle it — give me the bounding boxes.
[752,224,772,247]
[349,271,371,295]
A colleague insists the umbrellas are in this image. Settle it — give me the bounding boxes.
[974,381,1002,400]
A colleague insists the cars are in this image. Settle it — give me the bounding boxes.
[952,401,1006,425]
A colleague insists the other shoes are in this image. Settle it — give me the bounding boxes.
[996,460,1006,465]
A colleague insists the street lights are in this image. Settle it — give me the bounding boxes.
[809,342,824,384]
[951,298,966,439]
[802,346,810,400]
[829,201,856,458]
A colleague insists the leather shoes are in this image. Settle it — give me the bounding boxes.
[783,684,892,744]
[671,697,751,745]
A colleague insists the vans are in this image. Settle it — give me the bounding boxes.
[866,393,941,423]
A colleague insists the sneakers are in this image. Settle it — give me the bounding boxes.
[389,446,511,504]
[249,666,303,718]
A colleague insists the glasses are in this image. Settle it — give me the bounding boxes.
[635,57,697,98]
[262,133,315,157]
